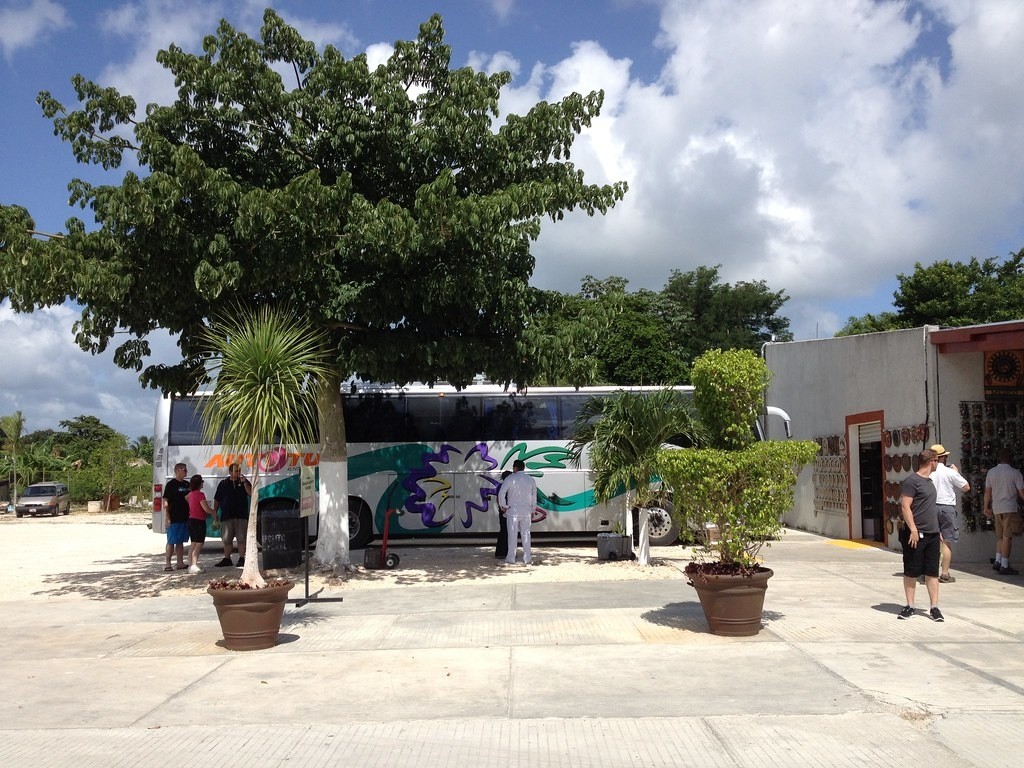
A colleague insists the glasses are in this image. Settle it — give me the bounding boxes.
[201,480,204,483]
[178,469,187,472]
[231,469,242,473]
[929,458,939,463]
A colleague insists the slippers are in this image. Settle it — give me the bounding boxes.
[176,564,189,569]
[163,567,175,571]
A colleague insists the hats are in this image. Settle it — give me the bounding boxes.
[930,444,950,456]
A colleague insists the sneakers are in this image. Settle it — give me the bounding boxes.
[215,558,233,567]
[236,557,244,567]
[187,564,206,574]
[938,573,956,583]
[930,607,945,622]
[898,605,917,620]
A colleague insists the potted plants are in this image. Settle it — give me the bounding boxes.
[189,291,342,651]
[654,436,820,640]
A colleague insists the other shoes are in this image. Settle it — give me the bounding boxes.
[999,566,1019,575]
[993,561,1001,571]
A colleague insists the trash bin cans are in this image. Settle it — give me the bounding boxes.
[260,506,302,571]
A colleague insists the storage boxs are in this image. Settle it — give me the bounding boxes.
[705,526,734,550]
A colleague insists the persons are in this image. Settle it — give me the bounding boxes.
[898,449,945,622]
[982,449,1024,575]
[213,463,252,567]
[495,460,537,563]
[163,463,191,570]
[929,444,970,583]
[183,474,213,574]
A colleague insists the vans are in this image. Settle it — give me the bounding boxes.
[14,482,71,518]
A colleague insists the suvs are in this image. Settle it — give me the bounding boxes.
[152,382,793,547]
